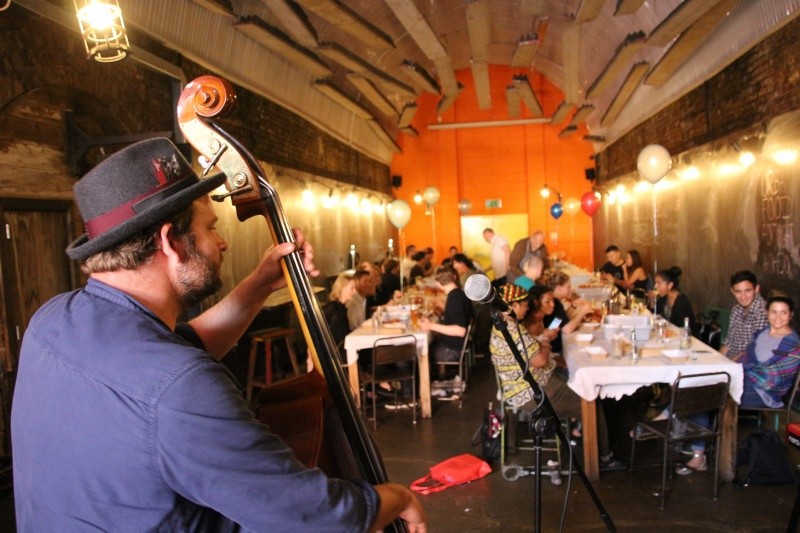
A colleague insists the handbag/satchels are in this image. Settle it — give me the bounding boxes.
[472,409,502,462]
[411,452,492,495]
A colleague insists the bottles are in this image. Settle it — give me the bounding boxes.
[680,317,691,351]
[629,330,638,365]
[401,307,409,332]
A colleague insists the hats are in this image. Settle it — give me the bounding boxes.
[499,284,528,303]
[66,137,227,260]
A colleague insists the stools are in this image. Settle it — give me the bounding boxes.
[246,327,302,400]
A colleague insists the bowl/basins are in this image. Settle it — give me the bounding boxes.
[661,349,688,362]
[574,334,594,345]
[585,346,607,359]
[580,322,600,331]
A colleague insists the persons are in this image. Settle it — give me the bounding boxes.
[9,139,429,533]
[331,227,800,477]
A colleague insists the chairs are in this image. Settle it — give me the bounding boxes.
[625,310,800,512]
[492,360,563,471]
[362,305,482,431]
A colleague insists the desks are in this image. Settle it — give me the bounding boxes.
[343,284,448,420]
[559,261,745,487]
[263,286,326,328]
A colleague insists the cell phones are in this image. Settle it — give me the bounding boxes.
[549,317,562,330]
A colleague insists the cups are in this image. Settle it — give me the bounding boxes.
[635,298,645,315]
[612,339,622,359]
[657,322,666,343]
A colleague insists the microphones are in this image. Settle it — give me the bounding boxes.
[464,273,516,319]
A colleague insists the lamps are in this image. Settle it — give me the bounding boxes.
[730,141,756,166]
[73,0,134,64]
[276,170,384,206]
[541,183,563,205]
[682,153,698,178]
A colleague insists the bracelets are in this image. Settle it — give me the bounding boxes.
[571,303,576,309]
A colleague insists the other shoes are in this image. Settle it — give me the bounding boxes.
[439,392,459,401]
[601,457,621,467]
[675,455,707,475]
[385,401,409,409]
[376,386,396,396]
[433,389,450,397]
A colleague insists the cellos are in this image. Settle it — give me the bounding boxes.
[176,73,409,533]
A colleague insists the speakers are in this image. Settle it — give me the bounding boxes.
[392,176,401,186]
[586,168,595,180]
